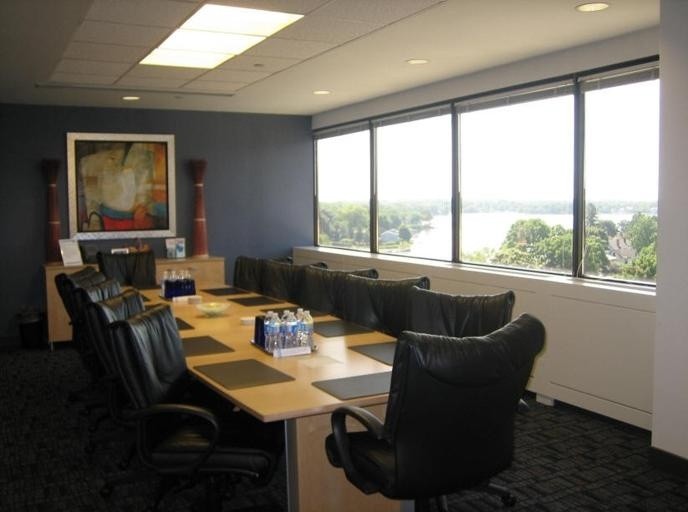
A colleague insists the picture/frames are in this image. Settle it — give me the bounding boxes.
[66,131,177,241]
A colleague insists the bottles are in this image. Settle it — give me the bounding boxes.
[161,268,196,301]
[265,306,315,354]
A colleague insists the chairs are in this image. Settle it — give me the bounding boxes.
[68,273,108,419]
[263,259,327,301]
[300,264,379,315]
[233,255,293,294]
[326,314,548,512]
[84,289,240,470]
[400,286,515,337]
[109,302,286,512]
[73,279,123,436]
[54,266,95,405]
[338,273,430,336]
[97,250,156,285]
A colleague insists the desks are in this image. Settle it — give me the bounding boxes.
[136,285,415,512]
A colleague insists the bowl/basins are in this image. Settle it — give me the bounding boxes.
[197,301,230,317]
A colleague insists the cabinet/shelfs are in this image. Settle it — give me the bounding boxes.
[42,255,225,352]
